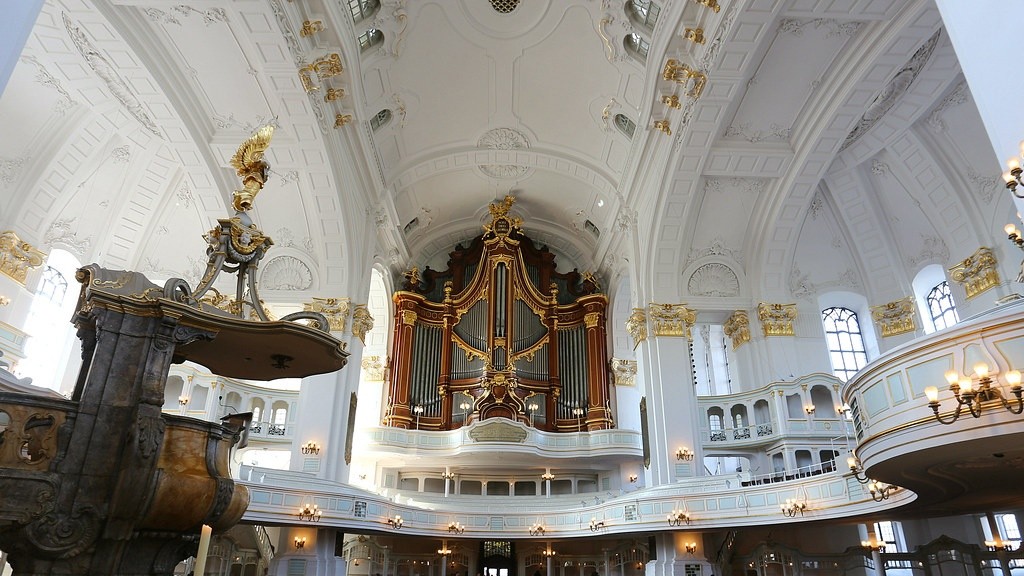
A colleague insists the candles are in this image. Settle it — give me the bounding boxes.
[194,524,212,576]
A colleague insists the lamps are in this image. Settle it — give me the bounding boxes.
[414,404,423,430]
[528,524,556,558]
[542,473,555,481]
[0,296,10,306]
[984,540,1012,554]
[630,475,637,483]
[460,400,470,426]
[574,408,584,432]
[924,361,1024,425]
[667,446,697,554]
[1002,139,1024,251]
[529,400,538,427]
[589,518,604,532]
[780,404,897,552]
[442,472,454,481]
[179,396,188,405]
[294,440,322,549]
[354,514,464,570]
